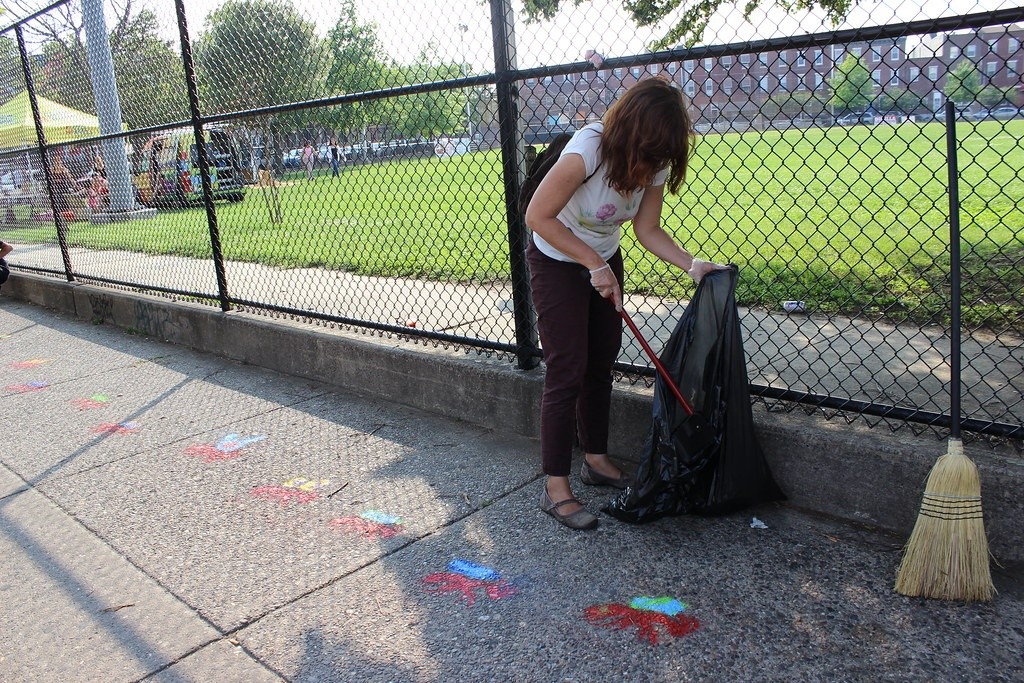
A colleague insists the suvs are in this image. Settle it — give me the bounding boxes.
[838,112,875,125]
[935,107,973,122]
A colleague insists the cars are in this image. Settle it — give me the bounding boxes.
[282,146,374,168]
[981,107,1021,119]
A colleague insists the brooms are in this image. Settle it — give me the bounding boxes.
[894,102,1004,601]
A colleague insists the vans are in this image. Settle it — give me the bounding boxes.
[251,146,283,171]
[137,125,259,207]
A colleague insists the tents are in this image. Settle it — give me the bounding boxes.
[0,90,136,211]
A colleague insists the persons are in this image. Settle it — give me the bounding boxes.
[41,150,76,211]
[302,140,320,182]
[0,240,13,260]
[84,155,107,209]
[327,138,347,179]
[525,78,732,532]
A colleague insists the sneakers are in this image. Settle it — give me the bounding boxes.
[539,484,599,530]
[581,462,637,489]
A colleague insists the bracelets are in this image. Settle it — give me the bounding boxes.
[589,263,610,274]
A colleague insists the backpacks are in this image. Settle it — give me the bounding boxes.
[517,133,572,217]
